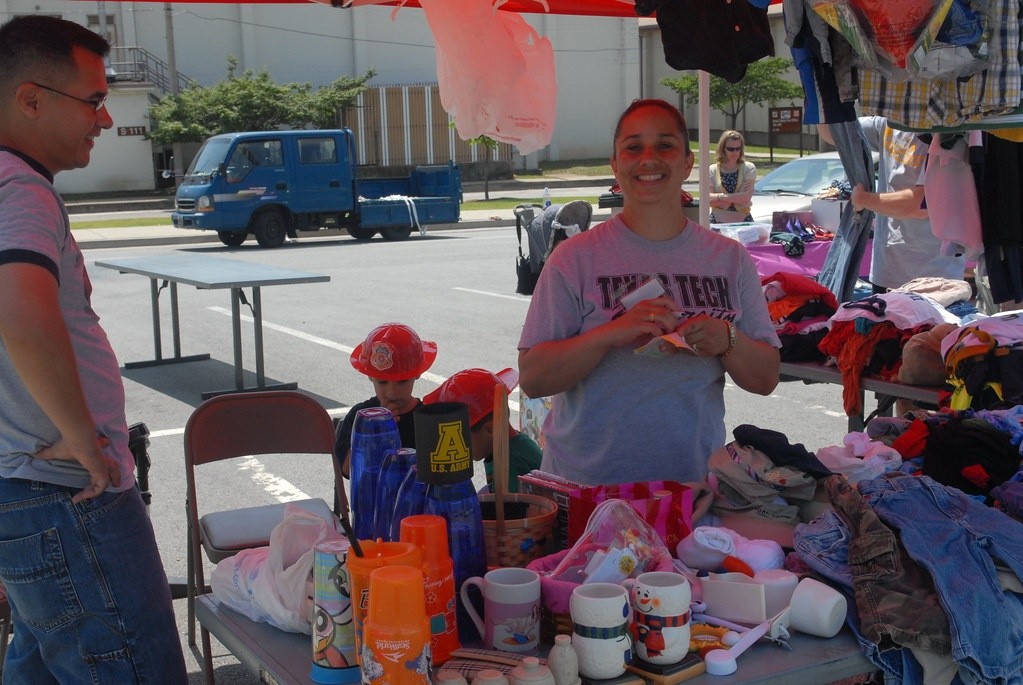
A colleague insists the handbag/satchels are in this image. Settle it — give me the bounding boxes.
[712,208,748,223]
[515,210,536,295]
[568,479,693,558]
[209,503,353,635]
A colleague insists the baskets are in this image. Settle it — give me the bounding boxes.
[476,382,558,568]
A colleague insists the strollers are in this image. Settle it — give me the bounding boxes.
[512,198,594,296]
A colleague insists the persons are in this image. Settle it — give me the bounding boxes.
[517,99,782,484]
[423,367,544,494]
[0,15,186,685]
[336,321,437,479]
[802,115,966,416]
[709,130,758,223]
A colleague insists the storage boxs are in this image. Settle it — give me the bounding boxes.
[710,221,773,245]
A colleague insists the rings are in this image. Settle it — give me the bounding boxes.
[649,312,655,322]
[692,344,699,351]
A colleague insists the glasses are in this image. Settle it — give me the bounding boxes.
[30,82,111,114]
[724,146,741,151]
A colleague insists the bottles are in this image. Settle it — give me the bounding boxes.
[308,406,484,685]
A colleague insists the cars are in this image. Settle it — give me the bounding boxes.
[742,150,881,225]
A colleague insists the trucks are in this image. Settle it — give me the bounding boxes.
[163,125,463,249]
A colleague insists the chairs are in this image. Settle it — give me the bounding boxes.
[185,393,350,685]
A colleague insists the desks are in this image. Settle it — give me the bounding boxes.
[97,250,333,399]
[192,592,880,685]
[779,359,946,436]
[744,240,874,275]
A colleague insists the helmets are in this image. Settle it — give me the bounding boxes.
[350,321,438,382]
[422,367,520,427]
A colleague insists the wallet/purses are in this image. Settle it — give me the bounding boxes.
[437,647,547,683]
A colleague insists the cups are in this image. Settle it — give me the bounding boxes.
[460,568,542,658]
[568,582,631,679]
[621,571,691,665]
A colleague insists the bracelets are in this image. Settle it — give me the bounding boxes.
[720,320,736,360]
[718,193,724,200]
